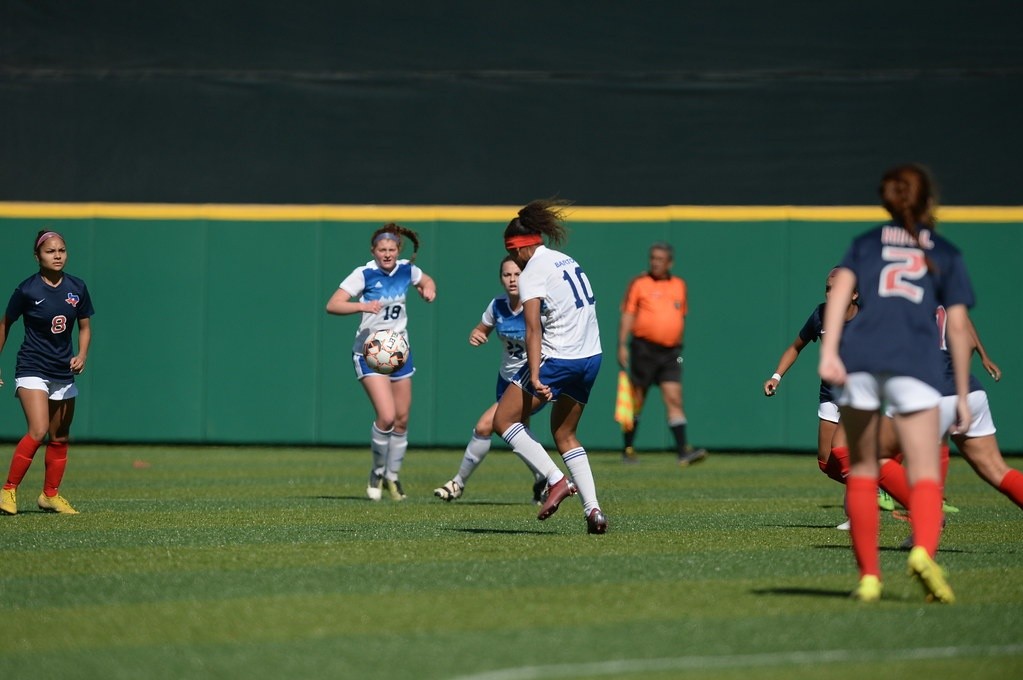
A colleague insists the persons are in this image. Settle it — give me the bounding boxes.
[0,230,96,514]
[760,167,1023,606]
[434,255,558,504]
[615,240,708,466]
[491,198,608,535]
[325,223,438,501]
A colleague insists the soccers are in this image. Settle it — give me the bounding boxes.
[362,328,409,375]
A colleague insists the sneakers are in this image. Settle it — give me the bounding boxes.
[906,545,955,605]
[433,480,464,501]
[878,487,895,511]
[893,508,910,521]
[367,470,383,500]
[383,477,405,501]
[538,475,577,520]
[901,519,947,550]
[836,520,851,530]
[38,491,79,514]
[849,574,882,601]
[531,478,549,505]
[0,489,17,514]
[941,497,959,513]
[584,508,608,534]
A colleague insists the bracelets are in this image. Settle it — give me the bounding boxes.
[772,373,782,381]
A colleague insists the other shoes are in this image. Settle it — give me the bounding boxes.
[679,448,707,467]
[623,448,642,464]
